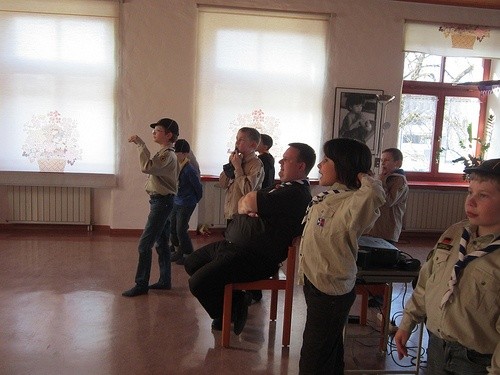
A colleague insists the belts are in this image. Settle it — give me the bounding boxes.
[223,239,233,248]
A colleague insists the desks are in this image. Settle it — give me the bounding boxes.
[342,268,425,375]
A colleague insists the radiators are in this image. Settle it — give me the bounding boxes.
[212,183,228,228]
[403,189,469,232]
[6,185,90,225]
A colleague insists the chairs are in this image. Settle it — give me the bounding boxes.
[222,246,297,350]
[354,282,391,351]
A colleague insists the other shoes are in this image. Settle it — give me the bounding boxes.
[368,299,383,308]
[234,291,253,336]
[171,253,181,262]
[176,257,186,264]
[211,319,222,331]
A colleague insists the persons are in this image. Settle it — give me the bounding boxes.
[253,134,275,187]
[122,118,182,295]
[168,138,203,265]
[296,138,385,374]
[186,143,316,330]
[359,148,408,308]
[395,158,500,375]
[220,127,265,307]
[340,96,372,135]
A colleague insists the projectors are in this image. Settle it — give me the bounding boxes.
[358,237,400,267]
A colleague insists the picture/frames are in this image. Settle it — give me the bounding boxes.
[333,87,385,155]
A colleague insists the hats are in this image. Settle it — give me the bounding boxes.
[150,118,179,135]
[463,158,500,174]
[172,139,190,153]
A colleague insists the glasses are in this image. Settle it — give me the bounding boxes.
[152,129,164,134]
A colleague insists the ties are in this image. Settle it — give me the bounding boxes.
[440,227,500,308]
[268,178,310,193]
[301,189,351,224]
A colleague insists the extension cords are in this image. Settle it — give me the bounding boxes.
[377,312,398,333]
